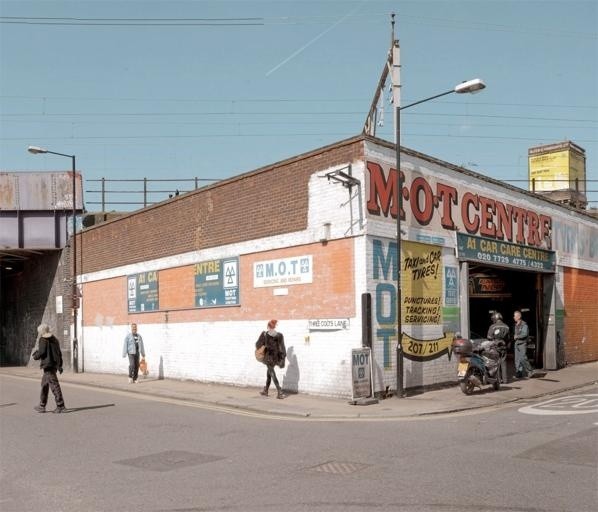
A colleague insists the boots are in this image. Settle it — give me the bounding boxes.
[275,388,283,399]
[258,386,269,395]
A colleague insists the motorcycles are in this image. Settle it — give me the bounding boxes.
[450,333,507,397]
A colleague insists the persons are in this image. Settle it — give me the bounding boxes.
[487,312,512,384]
[121,324,145,384]
[31,323,67,414]
[256,319,286,399]
[513,310,535,380]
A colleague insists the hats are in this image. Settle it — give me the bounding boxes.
[36,324,52,338]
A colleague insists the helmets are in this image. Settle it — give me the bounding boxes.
[491,312,503,323]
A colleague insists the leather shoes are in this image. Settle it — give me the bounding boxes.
[51,404,66,415]
[34,405,46,413]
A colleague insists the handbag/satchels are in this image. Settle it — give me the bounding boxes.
[138,358,148,375]
[254,331,267,362]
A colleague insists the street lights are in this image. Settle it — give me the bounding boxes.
[393,77,488,401]
[27,145,81,373]
